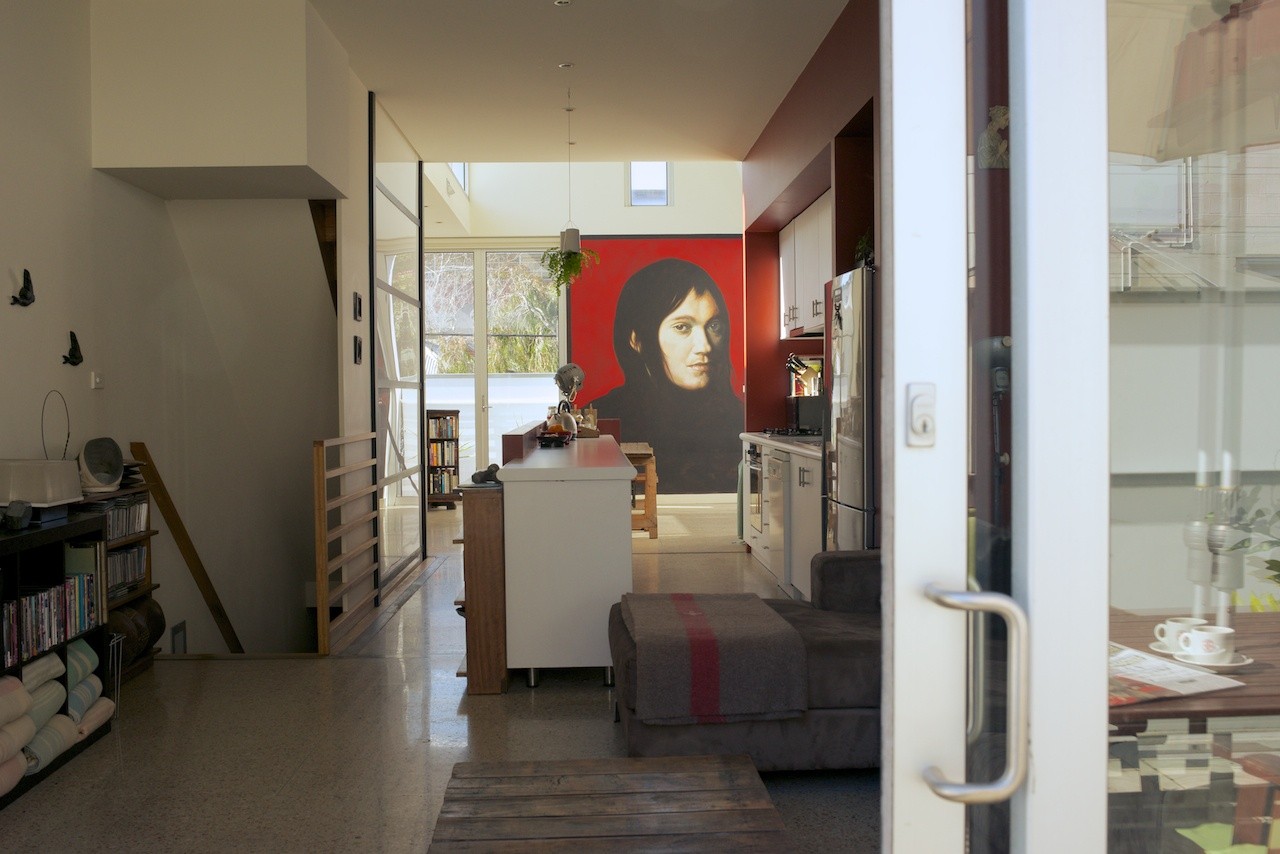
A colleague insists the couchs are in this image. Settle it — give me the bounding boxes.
[609,550,882,772]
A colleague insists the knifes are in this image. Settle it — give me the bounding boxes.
[784,352,807,376]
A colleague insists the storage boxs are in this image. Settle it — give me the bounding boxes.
[0,459,84,508]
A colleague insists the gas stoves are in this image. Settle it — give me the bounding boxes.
[758,426,822,439]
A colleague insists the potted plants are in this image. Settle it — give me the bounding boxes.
[540,229,600,296]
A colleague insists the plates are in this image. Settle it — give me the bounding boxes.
[1149,641,1187,654]
[1173,652,1254,674]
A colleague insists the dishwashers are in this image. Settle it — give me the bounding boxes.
[767,449,791,568]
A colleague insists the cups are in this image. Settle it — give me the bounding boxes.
[547,406,597,430]
[1153,617,1208,651]
[1179,626,1235,664]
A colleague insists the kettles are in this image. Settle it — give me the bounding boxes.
[546,400,578,439]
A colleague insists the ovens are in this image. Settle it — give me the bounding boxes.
[748,441,762,533]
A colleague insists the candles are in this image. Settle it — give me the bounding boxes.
[1196,450,1208,488]
[1220,451,1234,489]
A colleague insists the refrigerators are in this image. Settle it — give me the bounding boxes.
[825,267,873,553]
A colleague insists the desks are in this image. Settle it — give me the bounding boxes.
[1108,606,1279,734]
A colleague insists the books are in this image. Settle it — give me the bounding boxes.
[430,468,458,495]
[0,573,97,670]
[429,417,459,439]
[86,493,148,540]
[429,441,457,466]
[106,545,146,601]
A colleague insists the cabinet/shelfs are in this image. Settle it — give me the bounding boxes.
[0,485,164,811]
[743,441,822,607]
[427,409,462,510]
[451,487,505,696]
[778,188,835,335]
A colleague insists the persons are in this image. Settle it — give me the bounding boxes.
[578,259,748,494]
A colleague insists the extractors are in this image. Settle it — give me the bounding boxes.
[790,325,824,338]
